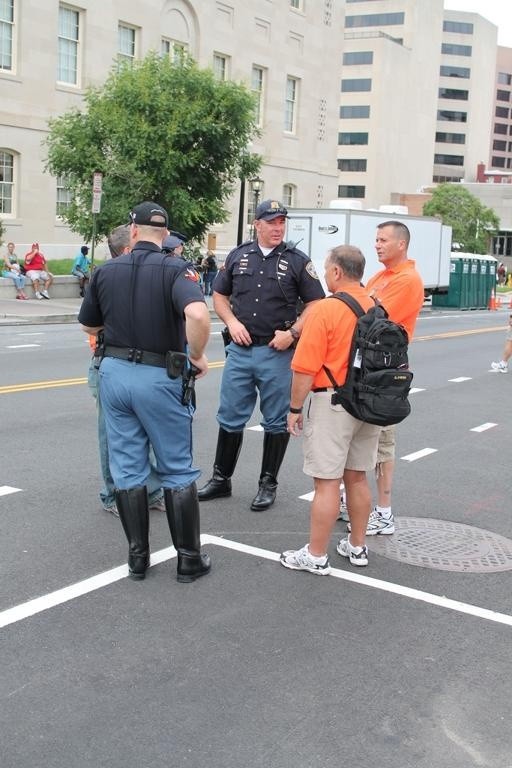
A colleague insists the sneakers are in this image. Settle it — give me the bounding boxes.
[103,504,119,517]
[490,362,507,373]
[279,495,395,575]
[16,289,50,299]
[149,497,166,512]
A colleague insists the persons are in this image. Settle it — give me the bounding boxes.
[163,230,189,260]
[204,250,219,296]
[24,242,53,299]
[497,263,506,289]
[337,221,423,536]
[197,257,204,292]
[280,246,384,575]
[86,225,168,516]
[196,199,325,510]
[1,243,28,300]
[491,295,512,370]
[77,199,211,584]
[72,246,97,297]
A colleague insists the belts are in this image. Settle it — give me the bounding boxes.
[251,334,273,347]
[311,388,335,392]
[101,346,165,368]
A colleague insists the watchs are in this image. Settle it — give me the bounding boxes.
[290,327,301,339]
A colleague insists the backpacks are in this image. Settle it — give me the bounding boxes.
[323,292,413,426]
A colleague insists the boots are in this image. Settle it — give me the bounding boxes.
[162,481,211,583]
[251,432,290,510]
[196,427,243,501]
[114,485,150,581]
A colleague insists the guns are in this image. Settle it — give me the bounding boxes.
[182,369,196,409]
[222,327,232,358]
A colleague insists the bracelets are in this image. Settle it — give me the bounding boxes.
[289,407,304,413]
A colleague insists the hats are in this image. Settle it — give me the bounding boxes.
[81,246,89,251]
[169,230,188,246]
[128,200,169,227]
[255,199,291,221]
[33,242,39,248]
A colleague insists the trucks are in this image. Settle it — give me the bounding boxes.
[251,207,453,301]
